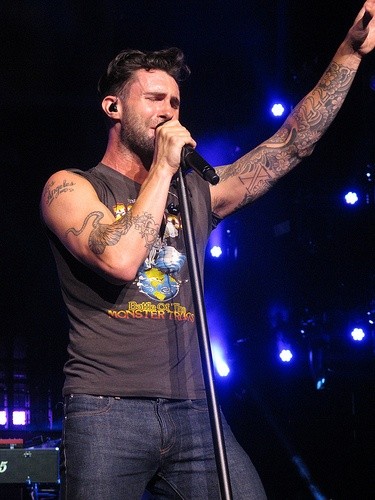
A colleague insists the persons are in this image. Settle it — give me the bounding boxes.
[42,1,374,500]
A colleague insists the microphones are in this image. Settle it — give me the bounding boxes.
[154,121,220,185]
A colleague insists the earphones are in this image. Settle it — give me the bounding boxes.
[109,101,118,112]
